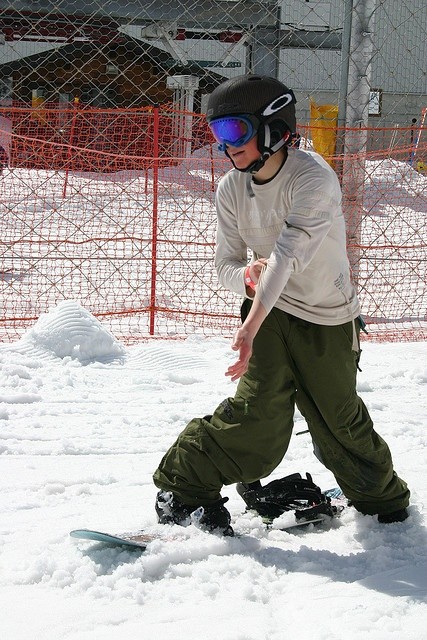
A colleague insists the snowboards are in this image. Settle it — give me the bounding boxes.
[69,486,352,548]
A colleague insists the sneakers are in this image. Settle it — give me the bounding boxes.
[156,490,231,528]
[363,510,409,523]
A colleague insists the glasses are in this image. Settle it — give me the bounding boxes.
[208,88,297,148]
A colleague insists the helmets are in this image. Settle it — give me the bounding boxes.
[207,75,296,133]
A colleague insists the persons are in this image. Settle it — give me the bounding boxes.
[150,74,410,536]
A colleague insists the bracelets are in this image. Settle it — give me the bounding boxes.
[246,266,257,291]
